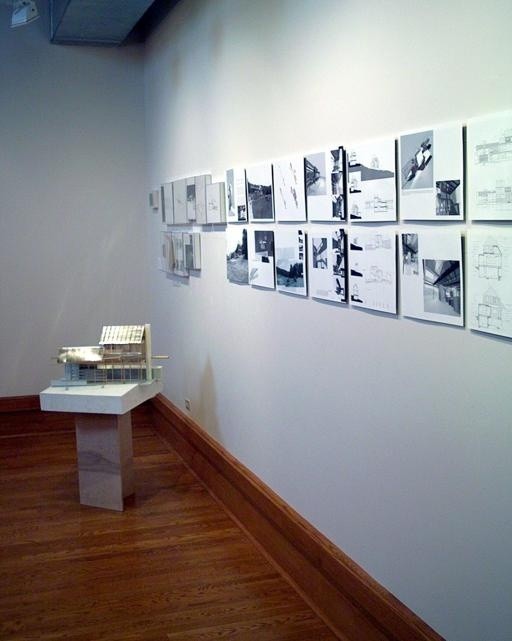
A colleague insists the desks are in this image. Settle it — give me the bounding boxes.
[40,377,164,513]
